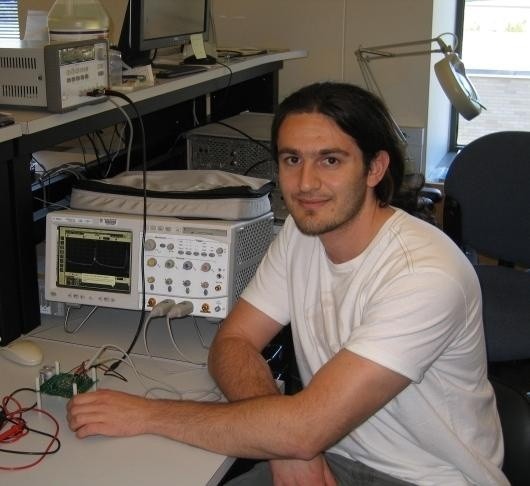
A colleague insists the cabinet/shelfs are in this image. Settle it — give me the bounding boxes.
[0,48,316,343]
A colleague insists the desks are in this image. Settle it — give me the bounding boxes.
[0,304,300,486]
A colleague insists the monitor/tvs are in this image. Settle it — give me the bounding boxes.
[118,0,208,78]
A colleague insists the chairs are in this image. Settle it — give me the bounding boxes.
[440,128,530,368]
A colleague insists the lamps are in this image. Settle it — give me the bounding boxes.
[352,32,483,191]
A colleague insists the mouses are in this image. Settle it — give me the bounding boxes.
[184,53,217,65]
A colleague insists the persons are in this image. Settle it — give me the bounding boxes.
[66,82,511,486]
